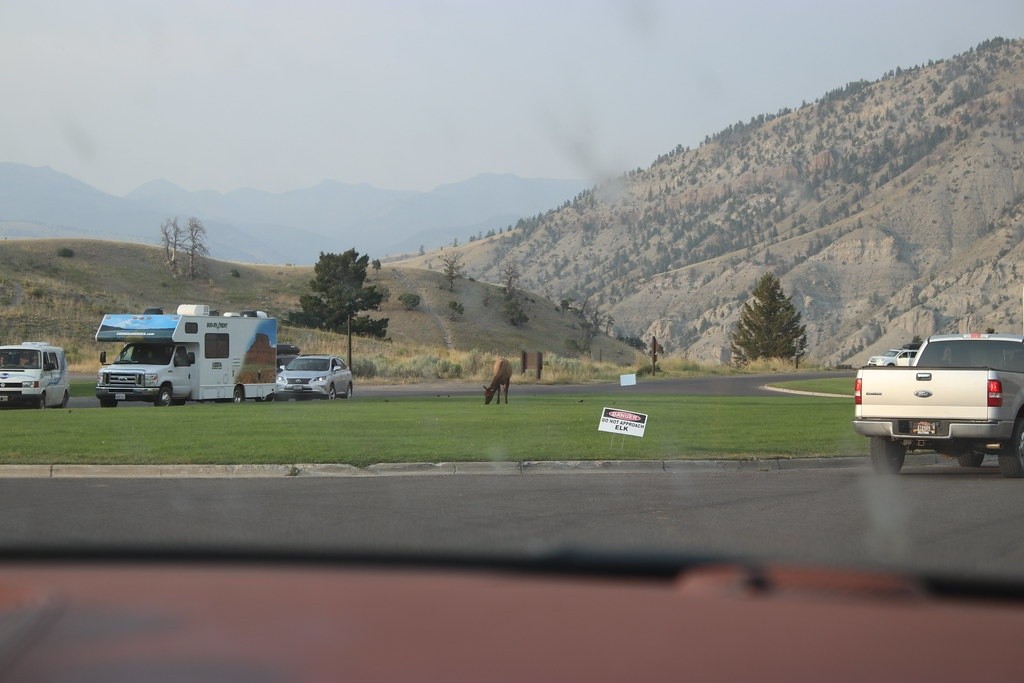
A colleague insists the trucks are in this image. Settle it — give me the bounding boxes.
[94,304,277,408]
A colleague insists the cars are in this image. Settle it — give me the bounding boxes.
[275,354,352,400]
[0,342,69,409]
[867,349,919,366]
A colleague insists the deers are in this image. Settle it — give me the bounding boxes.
[483,358,513,404]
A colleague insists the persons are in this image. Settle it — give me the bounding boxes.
[0,355,9,368]
[24,354,46,369]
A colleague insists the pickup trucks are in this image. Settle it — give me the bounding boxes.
[852,332,1024,478]
